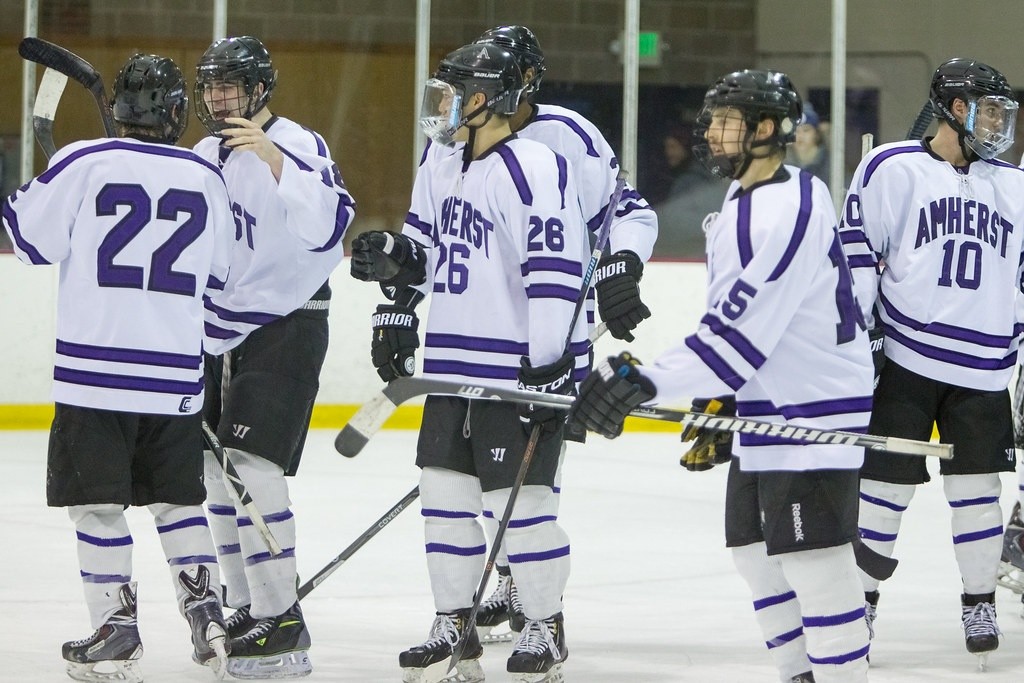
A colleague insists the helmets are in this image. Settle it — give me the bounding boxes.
[689,68,803,178]
[928,56,1013,159]
[435,42,522,149]
[193,35,275,138]
[109,53,187,142]
[473,25,546,96]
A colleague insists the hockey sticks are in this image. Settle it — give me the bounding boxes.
[331,371,959,463]
[856,131,874,162]
[420,168,629,683]
[17,34,235,420]
[302,320,606,607]
[31,66,286,561]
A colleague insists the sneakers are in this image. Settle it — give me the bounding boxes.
[504,597,570,683]
[176,565,232,682]
[224,573,312,681]
[863,591,880,664]
[399,591,486,683]
[505,576,525,645]
[474,571,512,645]
[223,603,259,640]
[960,591,1004,673]
[62,581,144,683]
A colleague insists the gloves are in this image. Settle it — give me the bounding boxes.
[567,353,658,441]
[370,304,420,383]
[594,250,651,344]
[679,396,738,472]
[517,350,574,444]
[864,327,883,382]
[351,229,426,287]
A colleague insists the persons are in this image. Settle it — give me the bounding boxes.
[834,59,1023,671]
[188,38,358,682]
[372,26,657,646]
[789,100,830,191]
[573,70,879,683]
[350,41,592,682]
[645,122,728,261]
[0,51,233,683]
